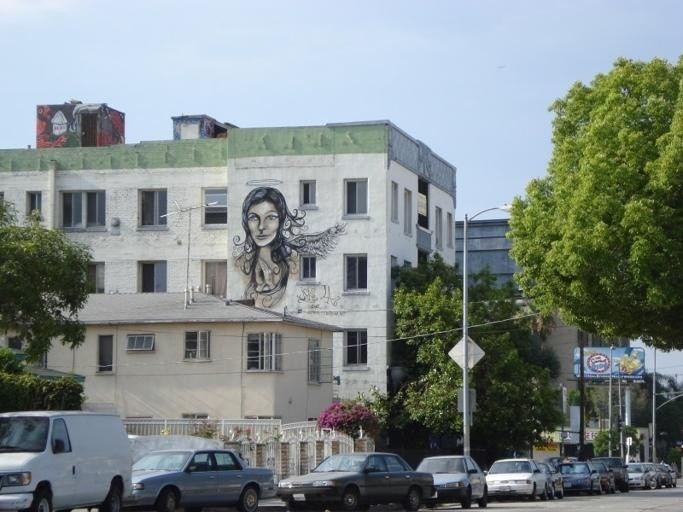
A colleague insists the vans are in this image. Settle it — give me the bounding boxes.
[0,411,132,512]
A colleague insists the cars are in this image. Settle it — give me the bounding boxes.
[122,449,273,512]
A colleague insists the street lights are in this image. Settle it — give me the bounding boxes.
[464,204,513,455]
[608,345,624,457]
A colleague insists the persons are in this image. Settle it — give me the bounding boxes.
[242,187,286,293]
